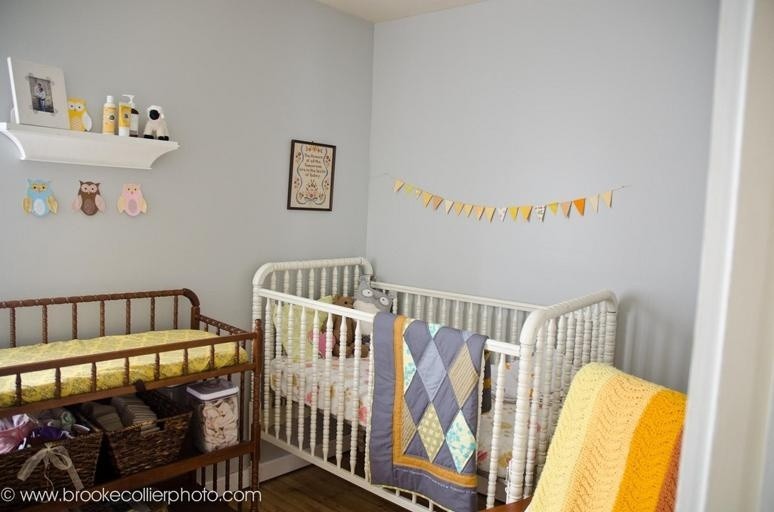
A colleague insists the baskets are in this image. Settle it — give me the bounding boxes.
[0,411,104,506]
[81,389,194,479]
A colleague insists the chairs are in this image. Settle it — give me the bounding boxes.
[474,361,687,512]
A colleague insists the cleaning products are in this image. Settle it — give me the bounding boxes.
[102,95,117,133]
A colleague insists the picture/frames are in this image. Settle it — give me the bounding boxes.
[7,57,70,130]
[287,139,336,211]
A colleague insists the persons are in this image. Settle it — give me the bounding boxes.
[34,83,46,111]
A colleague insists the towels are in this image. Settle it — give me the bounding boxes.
[525,361,687,512]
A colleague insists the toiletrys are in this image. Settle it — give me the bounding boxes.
[103,95,138,137]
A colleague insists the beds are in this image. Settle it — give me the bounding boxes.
[248,257,619,512]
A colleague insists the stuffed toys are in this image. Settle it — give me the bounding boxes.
[321,295,368,358]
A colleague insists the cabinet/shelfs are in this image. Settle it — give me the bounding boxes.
[0,289,263,512]
